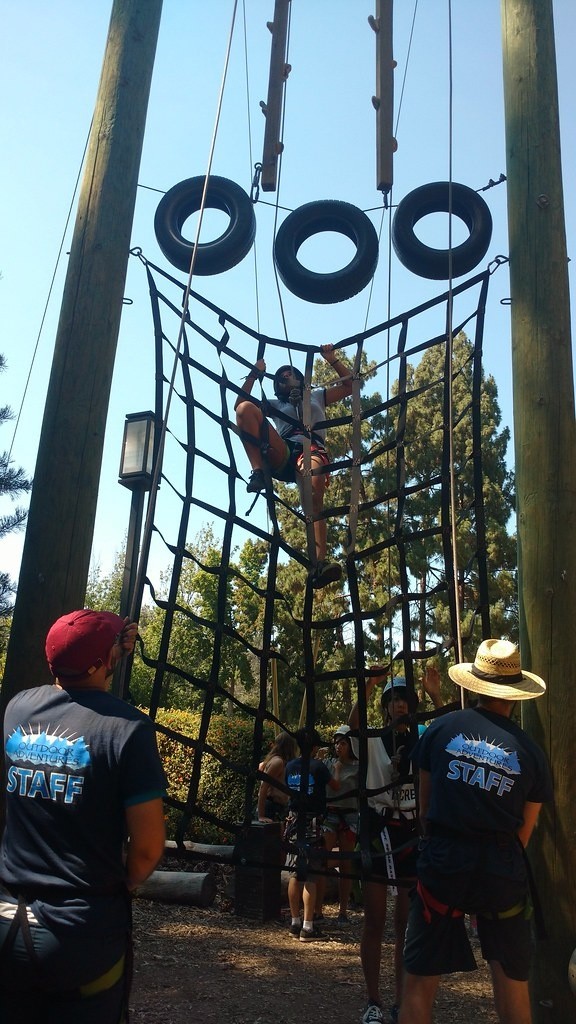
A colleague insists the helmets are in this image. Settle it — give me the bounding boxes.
[380,676,420,714]
[274,365,305,400]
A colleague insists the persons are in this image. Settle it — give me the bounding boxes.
[348,664,445,1024]
[397,639,552,1024]
[258,725,366,942]
[234,344,353,590]
[0,609,171,1024]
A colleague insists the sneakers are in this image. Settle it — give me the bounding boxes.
[300,927,329,942]
[287,924,303,938]
[389,1007,401,1024]
[362,1001,383,1024]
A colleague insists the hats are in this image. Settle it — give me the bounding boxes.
[333,724,359,759]
[448,639,546,701]
[45,610,125,677]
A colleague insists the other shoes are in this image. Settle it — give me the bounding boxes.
[313,913,325,924]
[247,469,273,492]
[337,914,350,926]
[311,559,342,589]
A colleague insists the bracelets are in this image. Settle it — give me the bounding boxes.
[330,359,339,367]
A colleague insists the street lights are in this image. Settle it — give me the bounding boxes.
[112,410,165,707]
